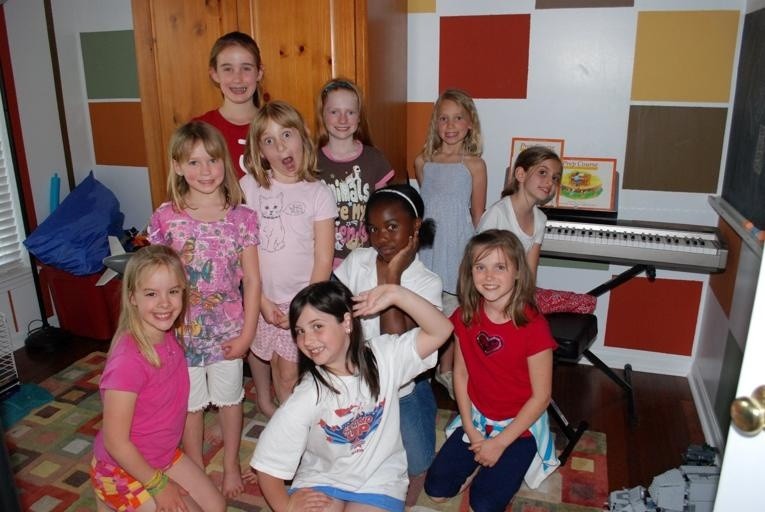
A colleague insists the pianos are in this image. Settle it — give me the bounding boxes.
[539,214,729,274]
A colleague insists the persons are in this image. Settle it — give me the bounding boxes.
[311,76,397,271]
[189,31,263,182]
[239,100,339,419]
[248,281,455,512]
[90,245,226,512]
[146,120,262,498]
[332,184,443,507]
[475,146,562,287]
[424,228,559,512]
[415,89,487,401]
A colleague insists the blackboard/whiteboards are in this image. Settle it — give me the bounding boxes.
[707,7,765,260]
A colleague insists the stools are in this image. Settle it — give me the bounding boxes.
[544,312,597,466]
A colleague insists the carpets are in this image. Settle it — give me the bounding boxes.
[0,350,609,512]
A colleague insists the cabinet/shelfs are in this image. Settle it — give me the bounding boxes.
[131,0,407,214]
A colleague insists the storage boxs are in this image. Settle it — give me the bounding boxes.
[40,265,112,341]
[105,276,124,336]
